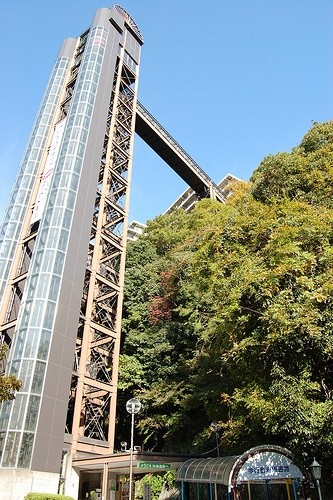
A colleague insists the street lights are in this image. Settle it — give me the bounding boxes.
[308,456,324,500]
